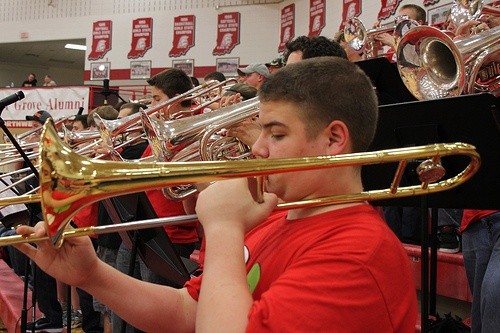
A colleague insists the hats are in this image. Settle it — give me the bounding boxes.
[237,63,269,78]
[265,58,285,68]
[26,110,53,125]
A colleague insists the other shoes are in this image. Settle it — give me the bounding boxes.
[438,231,462,253]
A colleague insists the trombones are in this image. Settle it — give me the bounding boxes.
[0,0,500,208]
[0,117,482,250]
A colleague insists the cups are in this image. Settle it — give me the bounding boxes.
[10,83,14,87]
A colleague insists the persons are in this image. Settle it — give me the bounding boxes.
[42,75,56,86]
[14,57,418,333]
[0,0,500,333]
[23,73,37,86]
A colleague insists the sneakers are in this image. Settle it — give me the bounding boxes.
[61,304,68,318]
[62,310,83,329]
[24,317,64,333]
